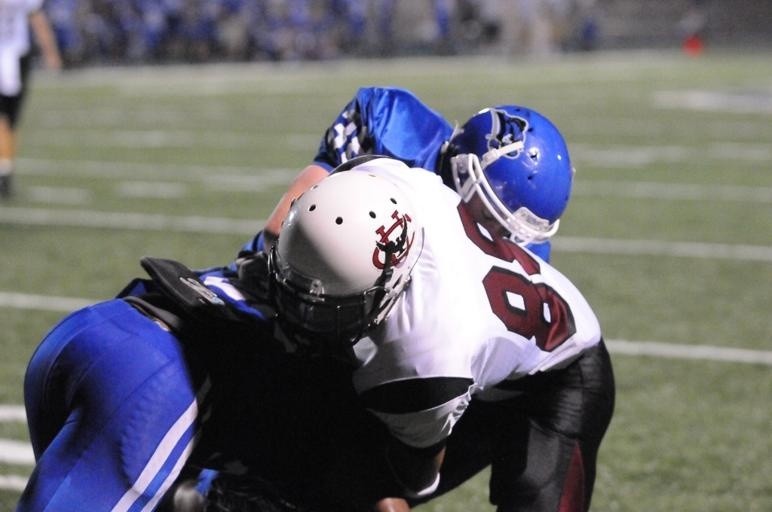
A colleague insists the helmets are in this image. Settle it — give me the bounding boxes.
[267,172,425,349]
[444,106,571,245]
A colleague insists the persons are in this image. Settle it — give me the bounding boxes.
[14,84,575,511]
[0,0,65,203]
[263,152,616,511]
